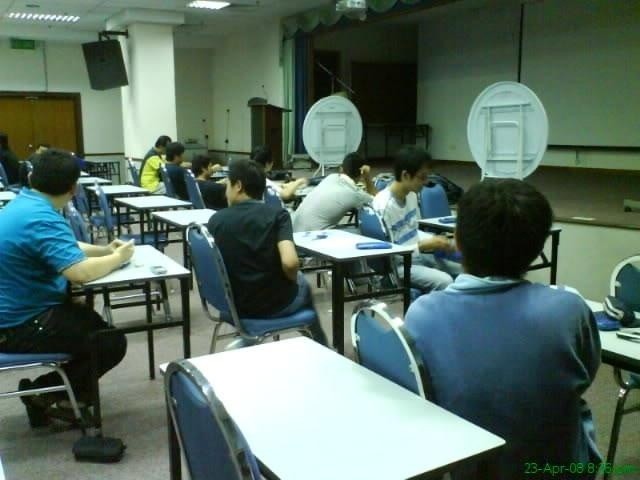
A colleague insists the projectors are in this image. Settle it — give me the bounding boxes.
[335,0,367,22]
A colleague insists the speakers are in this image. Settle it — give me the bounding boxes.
[81,39,129,91]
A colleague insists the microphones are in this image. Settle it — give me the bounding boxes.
[262,84,269,100]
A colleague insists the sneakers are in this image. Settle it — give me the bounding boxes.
[46,398,92,426]
[18,377,52,429]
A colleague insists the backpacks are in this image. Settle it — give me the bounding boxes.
[427,174,463,204]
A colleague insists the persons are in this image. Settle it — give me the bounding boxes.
[0,136,599,480]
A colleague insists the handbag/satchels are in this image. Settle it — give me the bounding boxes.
[73,438,122,462]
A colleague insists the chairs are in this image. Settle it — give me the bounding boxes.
[600,254,640,480]
[162,359,261,479]
[0,158,562,434]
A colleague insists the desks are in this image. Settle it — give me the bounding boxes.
[571,298,640,375]
[159,334,508,479]
[362,122,430,161]
